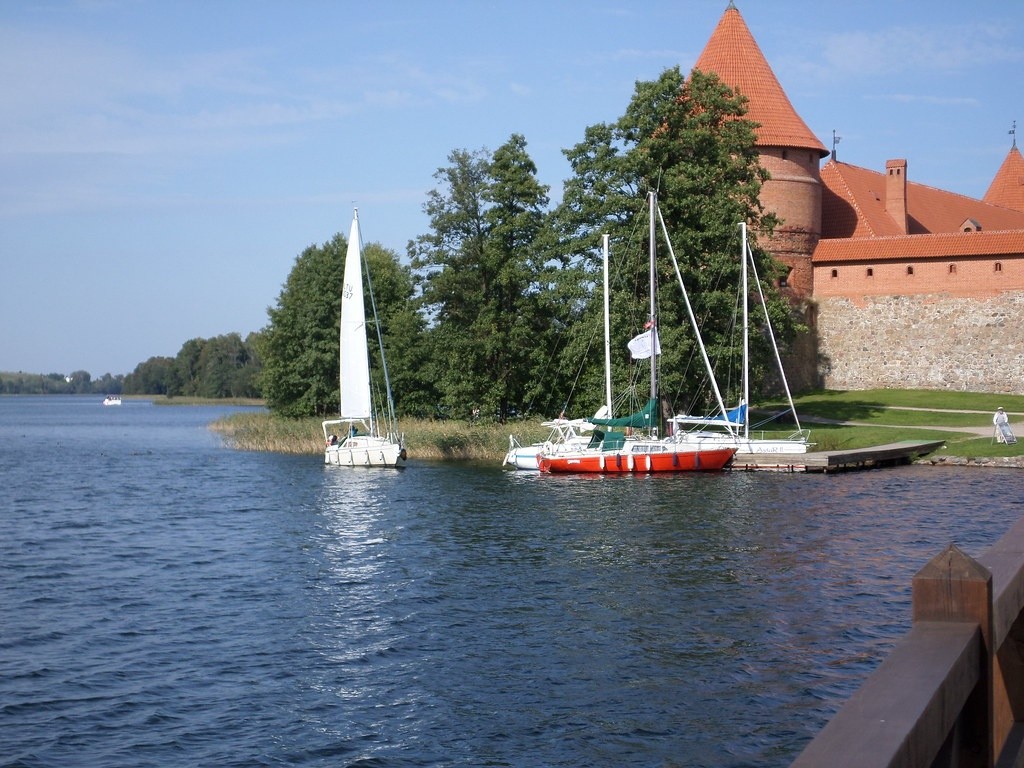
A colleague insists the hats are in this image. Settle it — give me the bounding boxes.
[998,406,1003,410]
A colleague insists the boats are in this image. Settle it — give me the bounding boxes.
[102,395,122,406]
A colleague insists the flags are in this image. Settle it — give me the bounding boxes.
[627,326,661,359]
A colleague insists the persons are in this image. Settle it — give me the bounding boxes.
[993,406,1009,444]
[348,424,358,435]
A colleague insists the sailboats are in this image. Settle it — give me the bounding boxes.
[503,188,818,475]
[319,206,405,467]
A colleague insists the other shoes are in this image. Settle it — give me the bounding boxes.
[998,441,1004,443]
